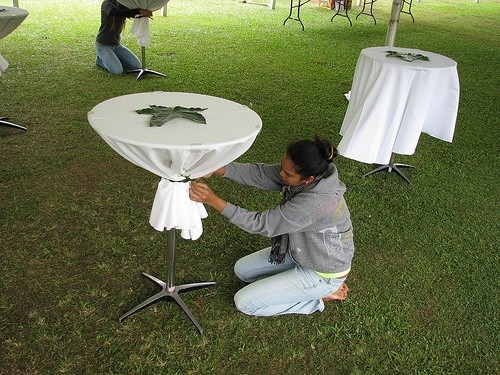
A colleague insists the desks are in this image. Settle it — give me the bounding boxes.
[337,47,460,185]
[86,91,262,335]
[116,0,170,81]
[0,5,29,130]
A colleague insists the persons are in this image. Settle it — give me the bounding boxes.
[189,139,355,316]
[95,0,153,74]
